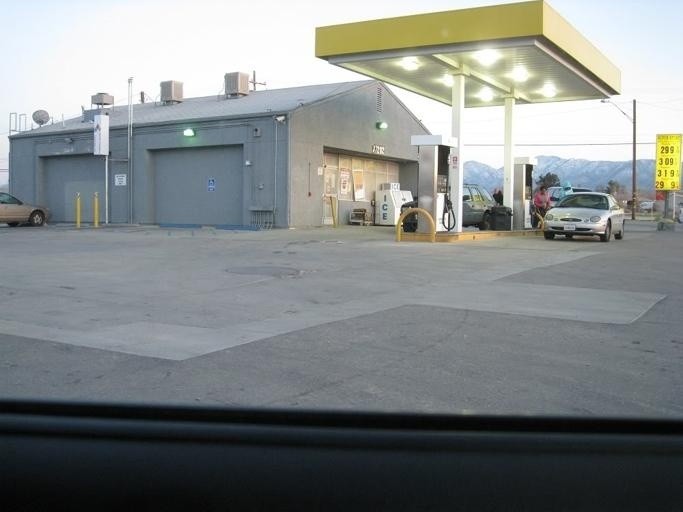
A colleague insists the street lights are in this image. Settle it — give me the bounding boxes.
[599,99,636,221]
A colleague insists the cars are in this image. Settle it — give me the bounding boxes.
[542,191,626,244]
[0,190,51,227]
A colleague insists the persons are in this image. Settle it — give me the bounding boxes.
[491,188,503,205]
[532,186,550,227]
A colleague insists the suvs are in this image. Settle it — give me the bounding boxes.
[400,182,499,234]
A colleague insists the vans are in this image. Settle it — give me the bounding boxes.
[543,186,591,208]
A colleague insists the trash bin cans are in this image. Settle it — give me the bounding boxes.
[491,206,513,231]
[400,202,418,232]
